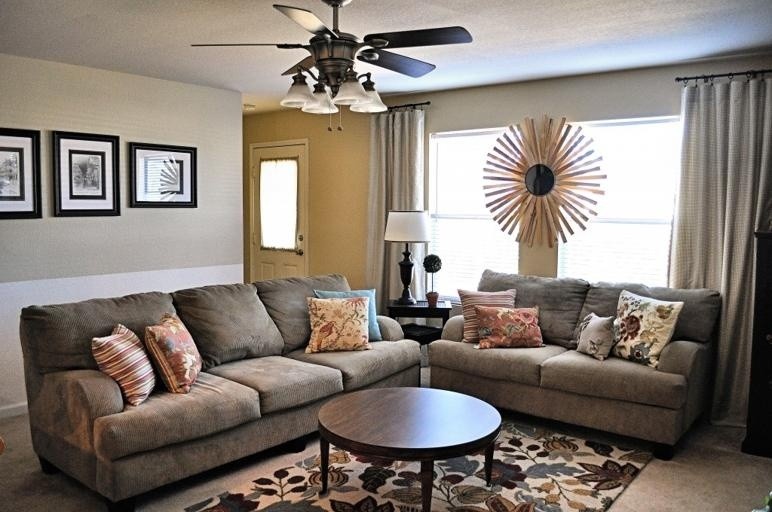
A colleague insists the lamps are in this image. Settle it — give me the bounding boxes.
[382,211,432,304]
[279,65,388,116]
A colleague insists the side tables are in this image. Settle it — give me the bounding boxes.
[386,300,453,343]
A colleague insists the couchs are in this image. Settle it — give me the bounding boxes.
[21,272,418,502]
[431,268,724,461]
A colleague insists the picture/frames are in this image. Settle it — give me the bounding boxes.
[0,126,197,219]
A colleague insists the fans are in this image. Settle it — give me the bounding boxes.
[191,0,473,133]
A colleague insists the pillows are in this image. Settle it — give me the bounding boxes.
[307,287,381,352]
[574,289,683,370]
[89,315,205,406]
[457,286,541,349]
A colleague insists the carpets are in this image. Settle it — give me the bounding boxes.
[179,416,655,512]
